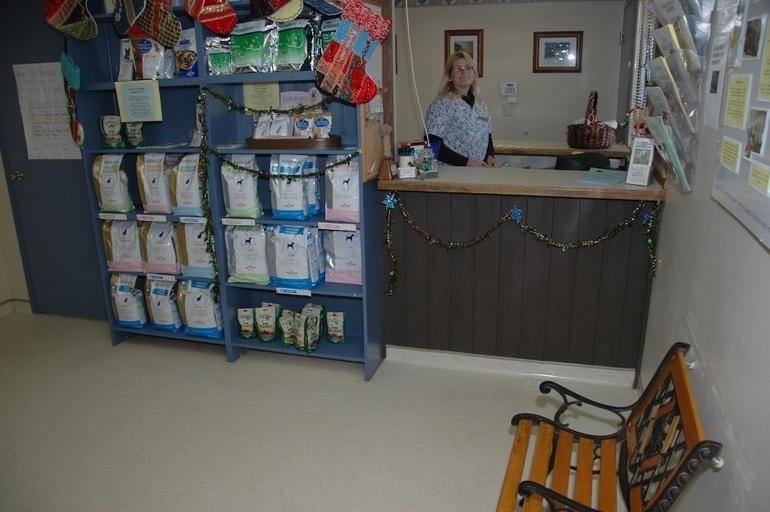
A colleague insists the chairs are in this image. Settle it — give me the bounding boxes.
[497,342,723,512]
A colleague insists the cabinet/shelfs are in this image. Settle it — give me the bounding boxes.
[65,1,387,382]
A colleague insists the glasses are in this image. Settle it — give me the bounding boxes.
[451,64,473,72]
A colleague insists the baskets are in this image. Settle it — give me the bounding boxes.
[565,89,616,150]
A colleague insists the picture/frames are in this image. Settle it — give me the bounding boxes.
[533,30,583,71]
[444,29,483,76]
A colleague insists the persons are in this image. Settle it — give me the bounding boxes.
[423,50,497,169]
